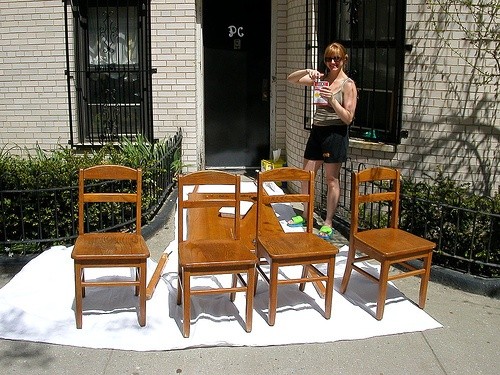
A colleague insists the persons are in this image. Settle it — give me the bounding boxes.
[287,43,358,240]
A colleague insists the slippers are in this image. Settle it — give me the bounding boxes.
[317,226,335,239]
[287,215,317,227]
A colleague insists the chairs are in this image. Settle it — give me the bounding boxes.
[338,166,437,321]
[70,163,151,327]
[177,169,260,338]
[253,166,339,327]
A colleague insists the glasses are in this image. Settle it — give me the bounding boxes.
[324,56,343,62]
